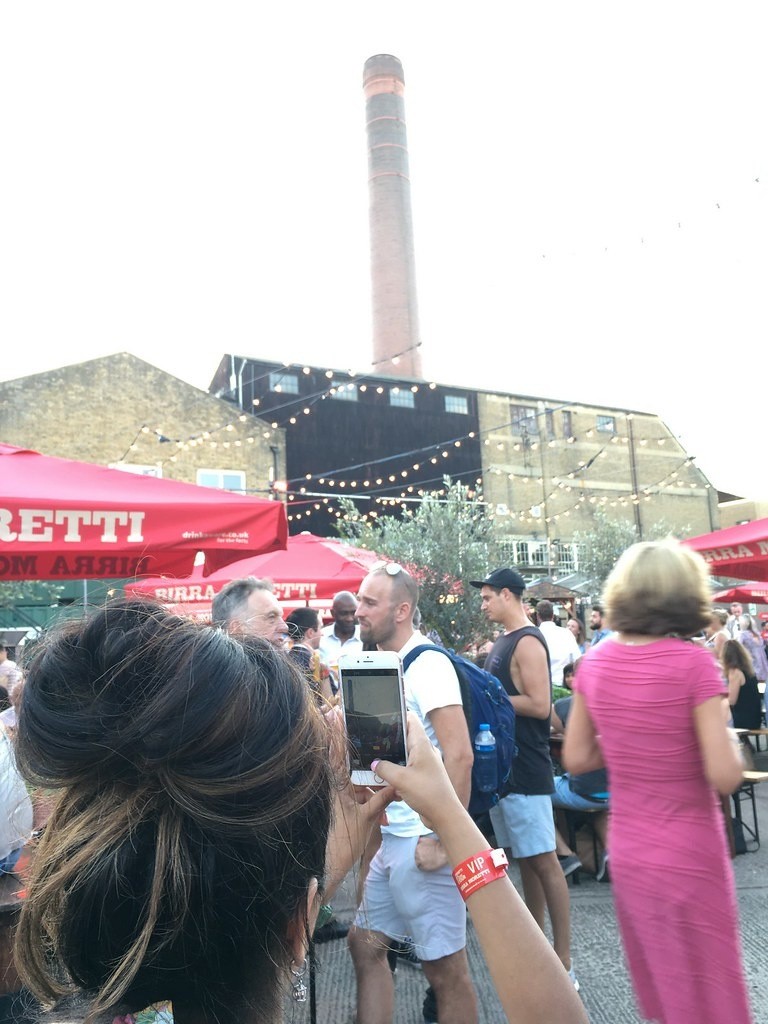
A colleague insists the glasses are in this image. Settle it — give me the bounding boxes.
[737,624,740,631]
[370,560,413,598]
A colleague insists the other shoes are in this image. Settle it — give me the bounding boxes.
[315,920,351,943]
[569,958,579,991]
[596,852,609,882]
[397,937,422,969]
[559,852,582,877]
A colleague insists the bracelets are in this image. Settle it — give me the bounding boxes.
[451,848,509,901]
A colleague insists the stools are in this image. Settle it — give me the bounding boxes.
[552,727,768,885]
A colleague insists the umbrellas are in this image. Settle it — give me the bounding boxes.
[125,530,464,625]
[681,516,768,604]
[0,442,289,581]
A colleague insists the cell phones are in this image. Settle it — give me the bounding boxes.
[338,651,409,786]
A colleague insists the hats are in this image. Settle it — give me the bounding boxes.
[469,568,525,588]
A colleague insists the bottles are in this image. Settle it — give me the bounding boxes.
[475,724,498,792]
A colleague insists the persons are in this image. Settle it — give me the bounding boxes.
[535,600,612,690]
[703,602,768,730]
[284,608,334,706]
[213,576,289,646]
[562,541,753,1024]
[314,591,363,666]
[347,561,478,1024]
[468,568,580,992]
[0,639,58,876]
[466,626,493,654]
[550,695,610,883]
[13,598,589,1024]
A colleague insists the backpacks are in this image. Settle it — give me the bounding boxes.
[402,645,518,814]
